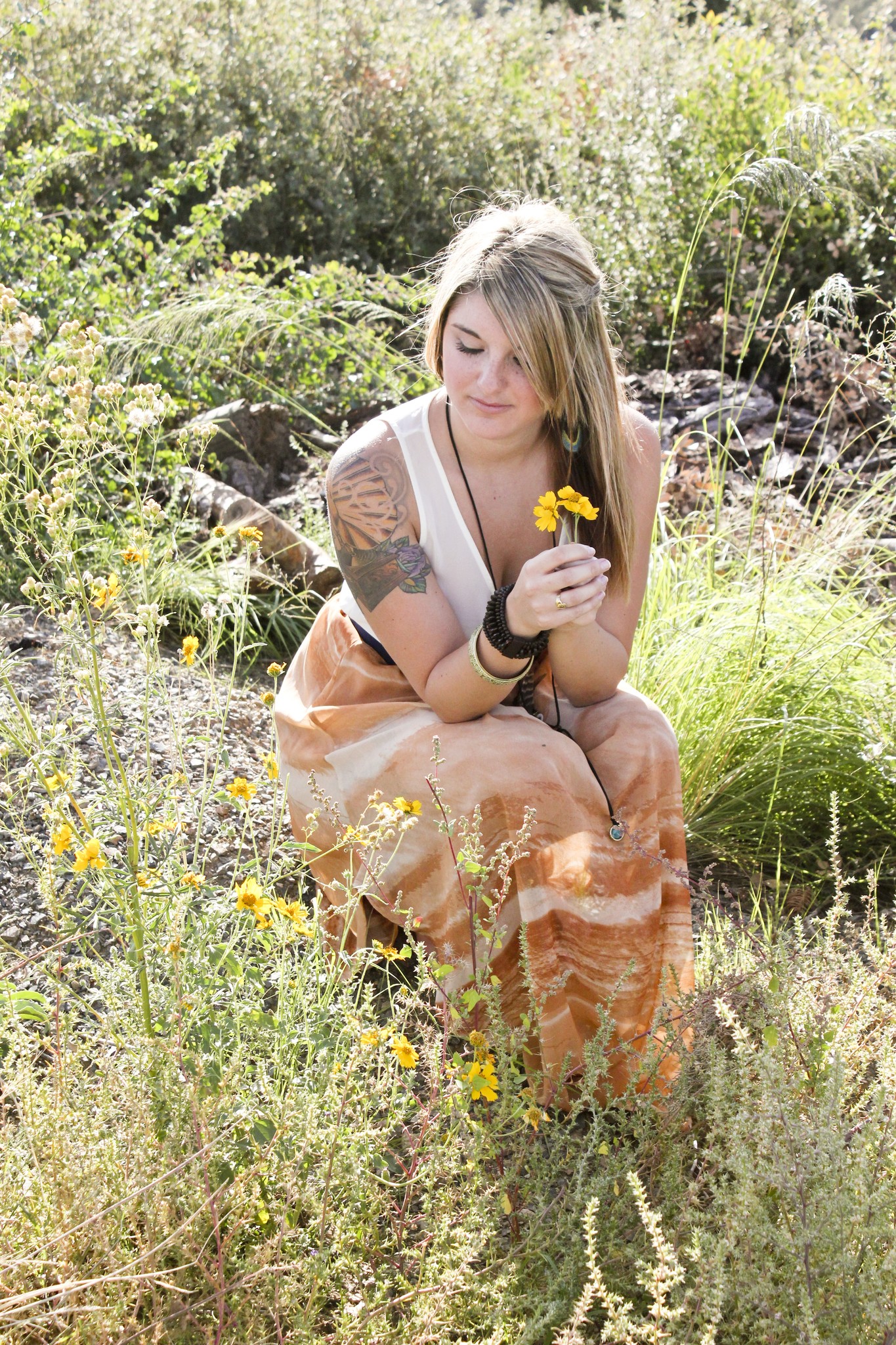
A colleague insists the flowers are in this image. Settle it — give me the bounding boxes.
[536,486,601,545]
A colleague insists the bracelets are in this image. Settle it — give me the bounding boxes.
[481,581,549,659]
[464,626,537,686]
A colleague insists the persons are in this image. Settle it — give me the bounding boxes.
[269,201,697,1146]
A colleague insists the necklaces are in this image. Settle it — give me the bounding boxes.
[444,394,623,842]
